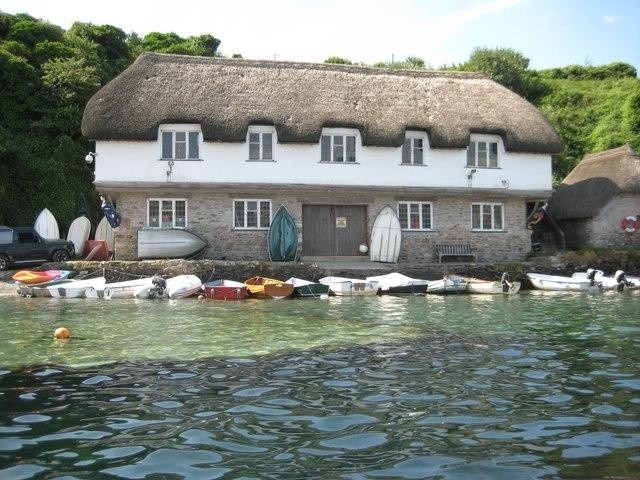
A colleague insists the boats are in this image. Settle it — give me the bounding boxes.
[267,204,298,262]
[137,227,209,261]
[369,205,402,264]
[34,207,116,257]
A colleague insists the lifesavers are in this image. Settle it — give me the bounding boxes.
[622,216,638,233]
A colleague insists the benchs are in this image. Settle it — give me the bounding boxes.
[435,243,480,265]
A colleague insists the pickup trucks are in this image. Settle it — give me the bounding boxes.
[0,225,76,272]
